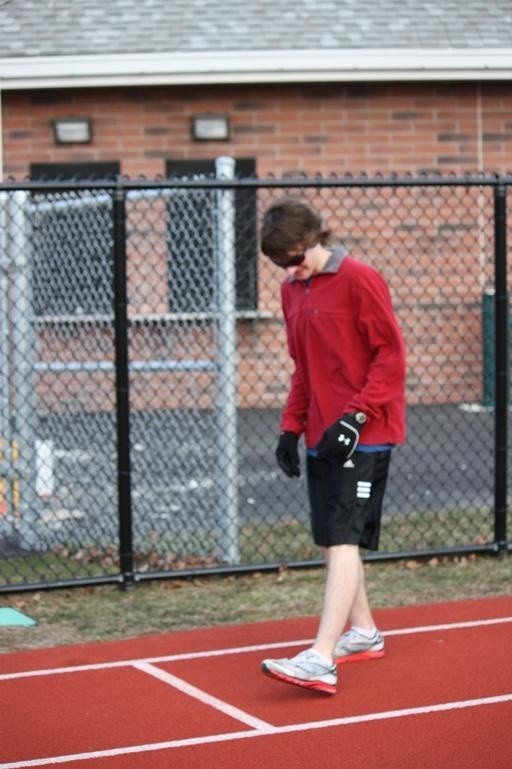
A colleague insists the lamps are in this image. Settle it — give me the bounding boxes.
[53,118,94,143]
[192,112,228,140]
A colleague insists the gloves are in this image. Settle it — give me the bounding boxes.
[313,410,362,461]
[275,431,303,478]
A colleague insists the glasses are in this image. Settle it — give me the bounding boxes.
[270,241,309,269]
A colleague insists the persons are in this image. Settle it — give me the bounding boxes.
[260,200,407,694]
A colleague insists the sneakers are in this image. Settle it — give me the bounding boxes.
[333,629,387,661]
[260,653,338,697]
